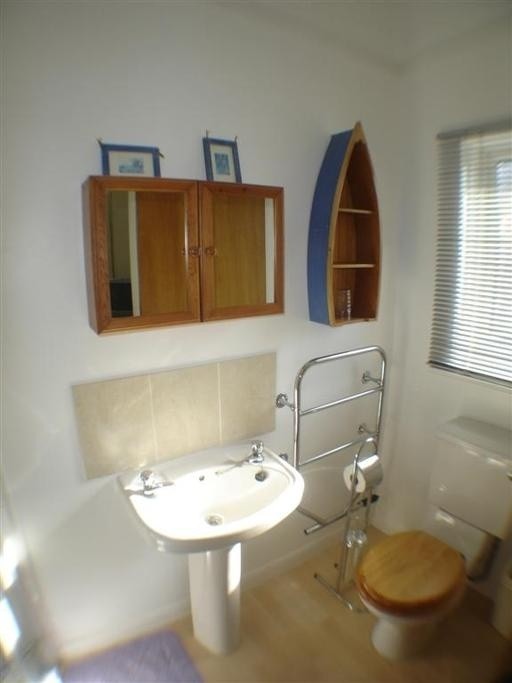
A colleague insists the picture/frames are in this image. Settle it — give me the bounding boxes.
[203,137,241,184]
[101,143,160,177]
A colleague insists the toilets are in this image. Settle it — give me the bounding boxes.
[350,416,511,661]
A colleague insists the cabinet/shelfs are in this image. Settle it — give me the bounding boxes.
[79,174,285,335]
[307,121,383,327]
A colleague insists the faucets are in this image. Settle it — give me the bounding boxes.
[141,470,176,496]
[244,439,265,466]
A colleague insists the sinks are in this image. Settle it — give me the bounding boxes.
[117,441,307,555]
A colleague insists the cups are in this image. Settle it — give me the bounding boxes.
[335,288,352,321]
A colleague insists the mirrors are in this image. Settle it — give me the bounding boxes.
[212,192,276,310]
[105,188,190,321]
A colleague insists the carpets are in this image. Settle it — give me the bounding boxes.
[61,630,204,683]
